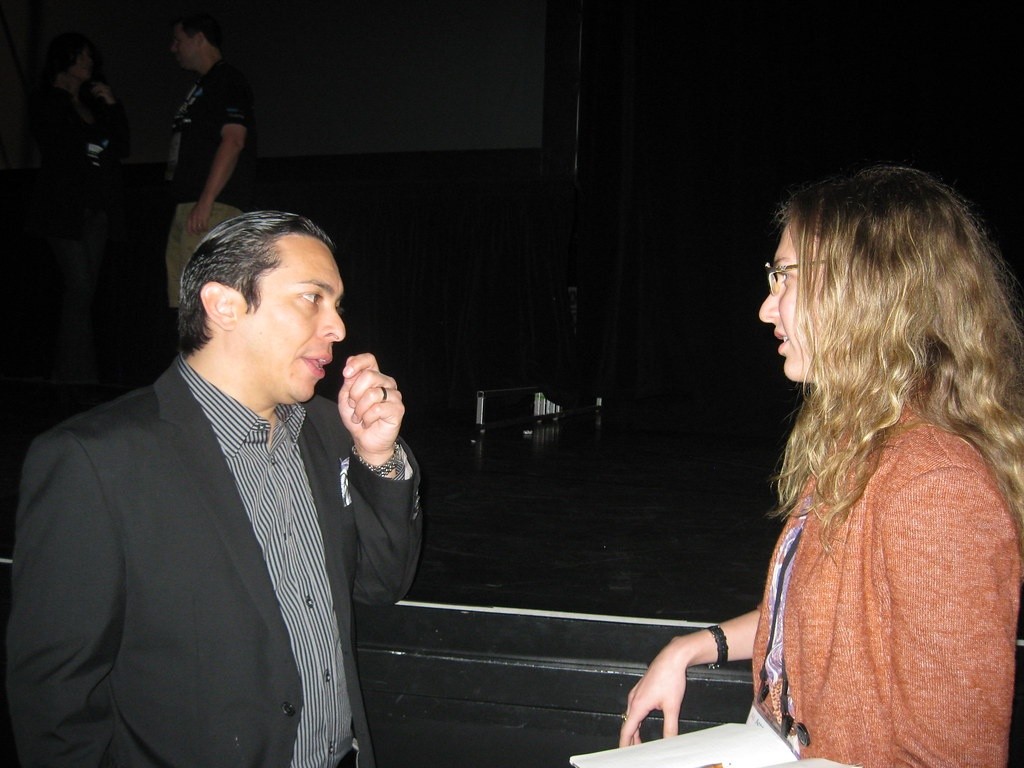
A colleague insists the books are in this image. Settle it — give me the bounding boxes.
[569,723,862,768]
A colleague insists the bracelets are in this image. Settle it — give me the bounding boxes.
[705,625,728,669]
[352,442,400,477]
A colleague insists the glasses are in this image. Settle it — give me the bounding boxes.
[765,260,826,296]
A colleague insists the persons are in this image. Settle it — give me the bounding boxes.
[26,32,130,382]
[6,211,422,768]
[161,14,257,307]
[619,167,1024,768]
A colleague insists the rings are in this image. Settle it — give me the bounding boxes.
[621,714,626,721]
[378,386,387,403]
[196,224,202,226]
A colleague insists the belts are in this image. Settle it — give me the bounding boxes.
[335,749,357,768]
